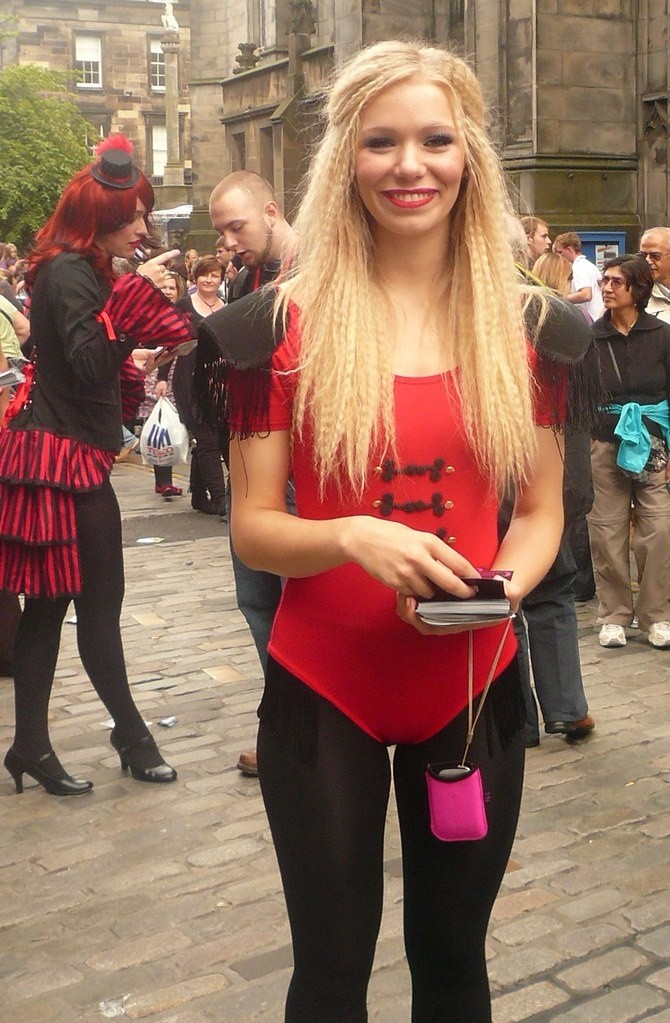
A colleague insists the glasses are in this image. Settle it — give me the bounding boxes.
[597,278,626,289]
[639,252,670,261]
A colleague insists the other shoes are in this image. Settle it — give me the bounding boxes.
[115,438,139,462]
[155,484,182,496]
[192,493,226,516]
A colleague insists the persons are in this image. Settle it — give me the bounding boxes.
[199,40,593,1023]
[0,134,670,799]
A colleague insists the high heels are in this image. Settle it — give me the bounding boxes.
[4,745,93,795]
[110,726,177,783]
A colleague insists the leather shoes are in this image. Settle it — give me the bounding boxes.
[237,747,257,774]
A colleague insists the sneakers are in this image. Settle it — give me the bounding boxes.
[648,621,670,647]
[525,737,540,749]
[544,714,595,734]
[628,615,638,628]
[599,623,626,647]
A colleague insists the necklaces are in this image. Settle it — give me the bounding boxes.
[610,319,638,337]
[197,293,218,312]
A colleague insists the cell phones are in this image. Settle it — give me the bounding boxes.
[152,346,168,358]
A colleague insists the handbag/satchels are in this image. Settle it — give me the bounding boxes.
[622,434,668,486]
